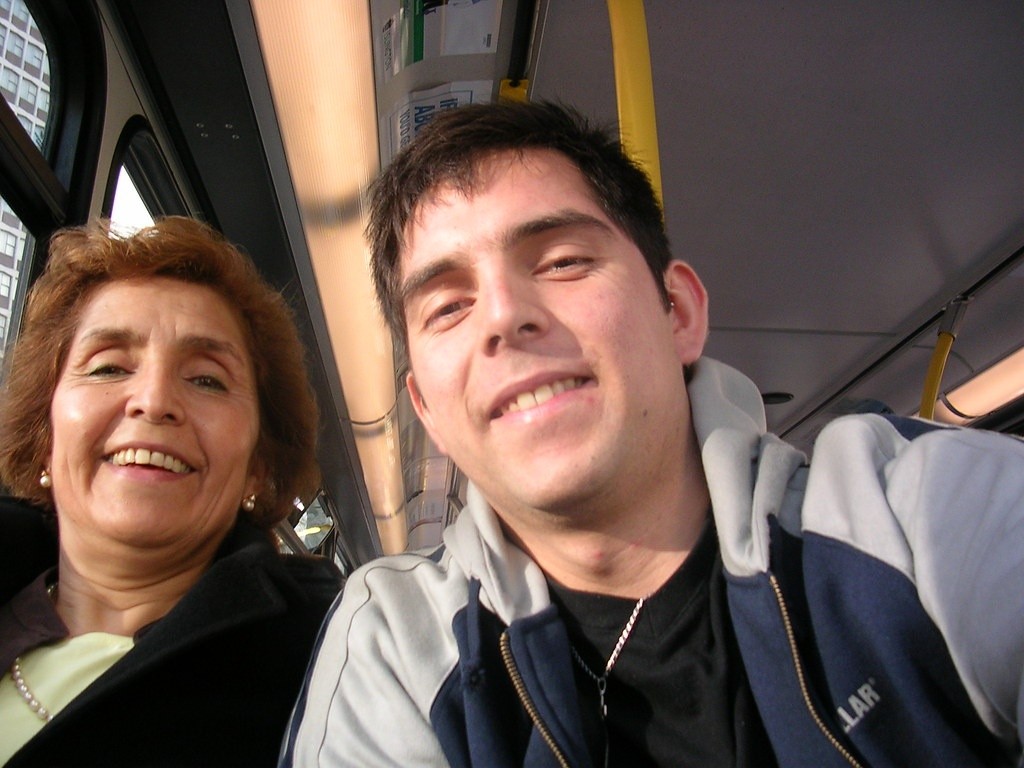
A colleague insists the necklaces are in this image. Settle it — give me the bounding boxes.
[11,582,56,722]
[570,593,654,720]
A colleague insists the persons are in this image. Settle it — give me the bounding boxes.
[278,100,1024,768]
[0,217,343,768]
